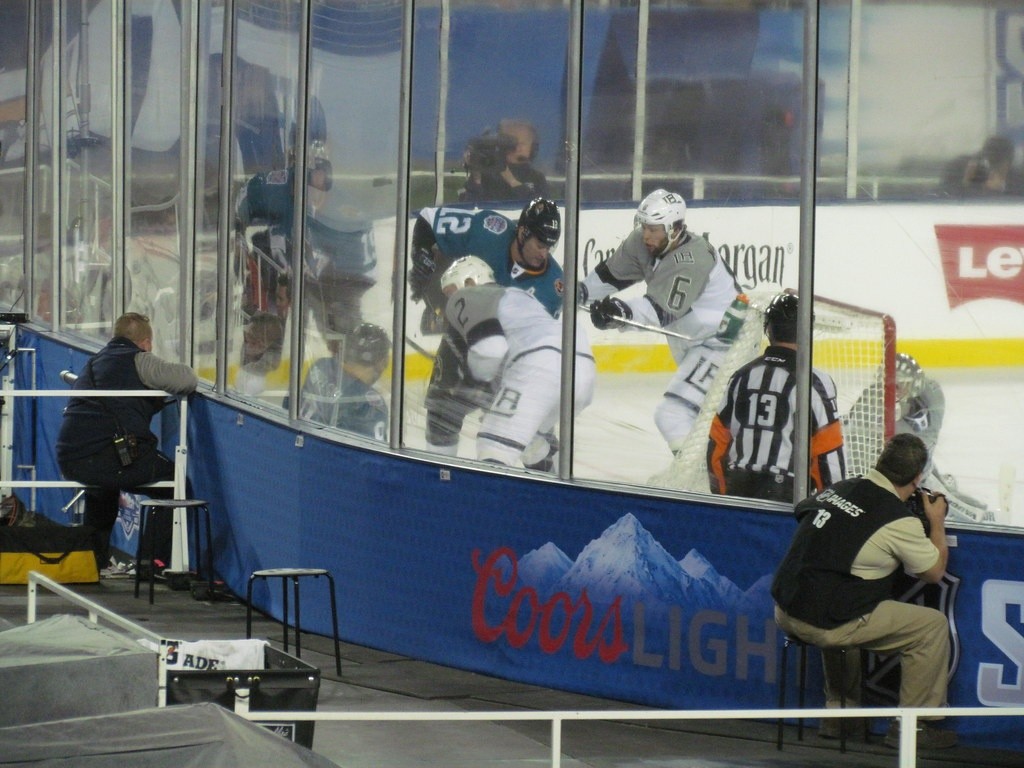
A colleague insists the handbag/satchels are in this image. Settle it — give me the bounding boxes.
[0,527,99,584]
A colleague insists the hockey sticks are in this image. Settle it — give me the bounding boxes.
[576,303,717,342]
[927,473,1007,526]
[422,291,467,364]
[405,335,436,363]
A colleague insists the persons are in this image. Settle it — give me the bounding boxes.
[408,197,565,456]
[941,136,1024,196]
[706,287,846,505]
[55,312,198,581]
[578,188,759,489]
[297,323,389,441]
[769,434,959,749]
[457,118,547,208]
[233,140,376,394]
[847,354,946,482]
[441,254,593,475]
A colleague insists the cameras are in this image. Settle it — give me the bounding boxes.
[906,486,949,521]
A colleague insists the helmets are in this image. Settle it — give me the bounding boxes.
[636,188,687,234]
[763,292,816,338]
[518,196,561,246]
[441,256,497,290]
[875,353,926,422]
[348,323,392,362]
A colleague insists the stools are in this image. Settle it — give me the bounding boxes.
[777,633,871,753]
[247,567,343,676]
[135,500,215,605]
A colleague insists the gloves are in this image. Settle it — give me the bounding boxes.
[448,373,490,417]
[590,295,633,330]
[408,245,437,305]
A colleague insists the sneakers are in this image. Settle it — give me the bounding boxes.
[817,718,872,738]
[885,721,957,750]
[142,567,167,581]
[98,562,129,578]
